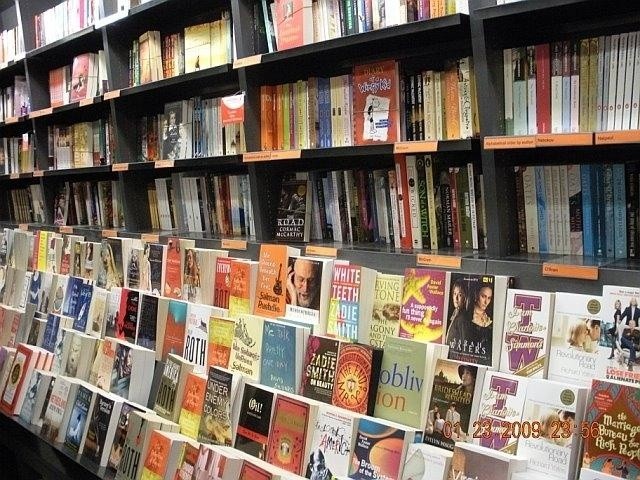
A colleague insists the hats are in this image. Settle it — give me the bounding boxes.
[457,364,478,380]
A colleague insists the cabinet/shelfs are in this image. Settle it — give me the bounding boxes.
[0,0,45,221]
[98,0,258,238]
[232,0,492,254]
[0,227,640,480]
[17,1,127,229]
[470,0,639,257]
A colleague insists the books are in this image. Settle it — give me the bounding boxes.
[2,228,640,479]
[127,13,256,238]
[1,0,125,233]
[494,1,640,259]
[252,0,486,251]
